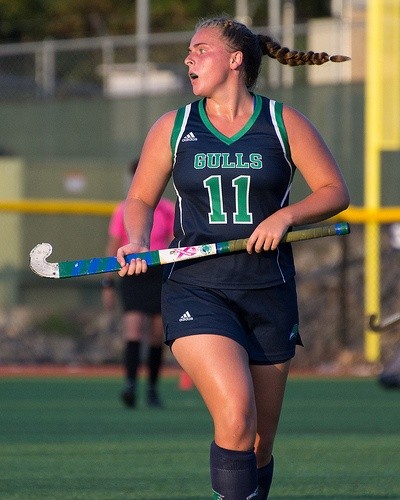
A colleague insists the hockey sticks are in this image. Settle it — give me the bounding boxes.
[27,219,351,280]
[362,314,400,332]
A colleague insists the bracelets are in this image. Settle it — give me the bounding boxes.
[100,278,115,290]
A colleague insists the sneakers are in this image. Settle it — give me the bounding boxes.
[121,386,163,410]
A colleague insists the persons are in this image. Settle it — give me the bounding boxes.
[99,158,176,412]
[117,15,350,500]
